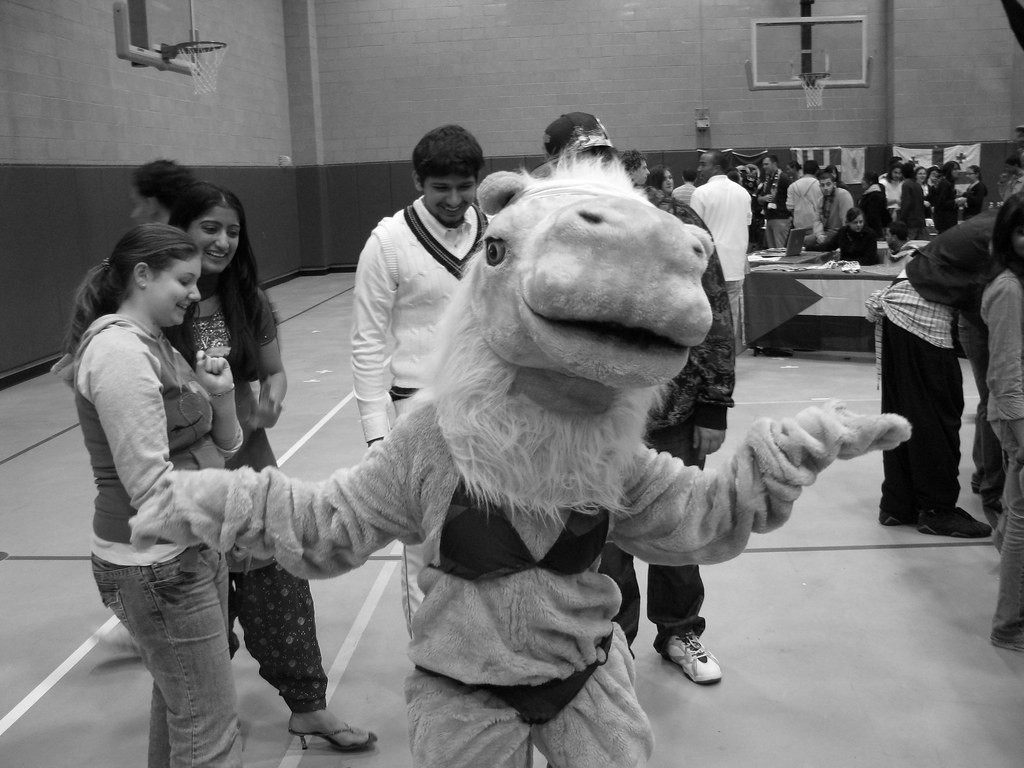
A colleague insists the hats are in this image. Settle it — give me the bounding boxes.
[530,111,614,179]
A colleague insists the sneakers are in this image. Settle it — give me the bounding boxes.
[983,497,1003,514]
[660,629,722,683]
[972,480,979,494]
[918,506,993,538]
[991,628,1024,652]
[880,505,918,526]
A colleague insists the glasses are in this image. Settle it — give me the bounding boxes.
[964,172,976,175]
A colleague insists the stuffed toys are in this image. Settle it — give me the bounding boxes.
[135,151,914,767]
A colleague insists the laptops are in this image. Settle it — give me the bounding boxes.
[763,228,806,258]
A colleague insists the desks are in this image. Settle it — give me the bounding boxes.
[735,241,901,358]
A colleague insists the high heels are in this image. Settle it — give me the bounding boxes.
[287,722,378,751]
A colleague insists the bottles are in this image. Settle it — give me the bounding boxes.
[988,201,1003,209]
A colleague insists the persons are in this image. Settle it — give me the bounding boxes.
[881,155,987,241]
[645,164,675,195]
[344,123,498,630]
[50,220,244,768]
[689,147,752,336]
[997,124,1024,204]
[734,154,855,247]
[804,207,879,265]
[540,110,742,687]
[672,169,698,206]
[883,220,908,252]
[621,147,652,186]
[864,201,993,540]
[122,157,379,751]
[979,190,1024,651]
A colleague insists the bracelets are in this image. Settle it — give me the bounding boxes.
[208,383,236,399]
